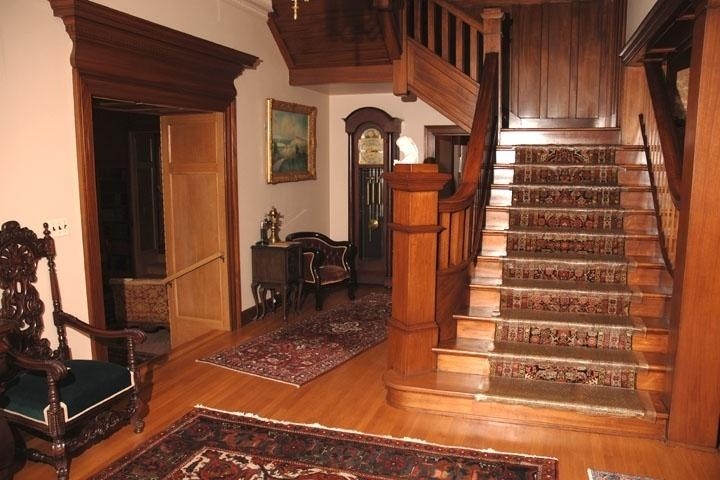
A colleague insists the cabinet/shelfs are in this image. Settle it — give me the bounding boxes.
[252,242,299,322]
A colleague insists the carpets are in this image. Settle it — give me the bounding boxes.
[194,292,393,387]
[74,405,559,479]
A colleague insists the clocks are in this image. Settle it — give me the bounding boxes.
[343,105,403,289]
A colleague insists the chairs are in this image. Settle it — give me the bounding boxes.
[110,271,169,333]
[0,221,146,478]
[287,232,358,311]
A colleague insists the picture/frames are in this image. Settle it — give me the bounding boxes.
[266,97,318,184]
